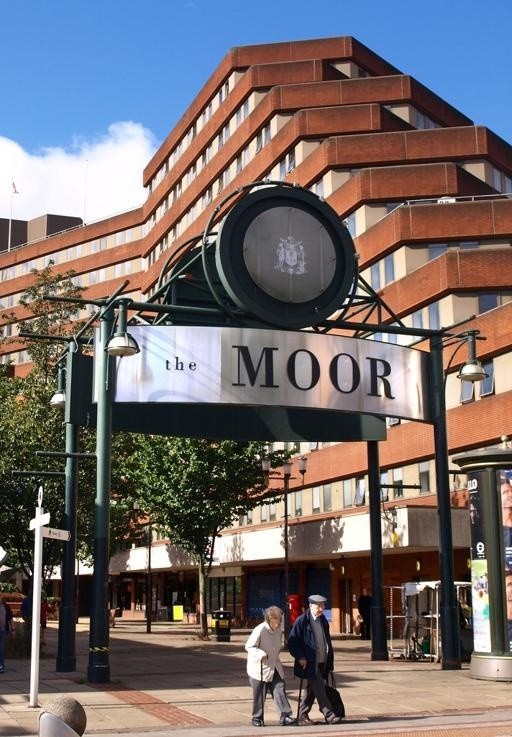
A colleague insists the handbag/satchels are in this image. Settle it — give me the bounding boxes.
[325,671,345,717]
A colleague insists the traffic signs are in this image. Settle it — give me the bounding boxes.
[28,513,71,541]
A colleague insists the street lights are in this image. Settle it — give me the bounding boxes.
[50,340,83,672]
[131,501,152,634]
[262,457,307,650]
[430,328,490,670]
[88,296,140,682]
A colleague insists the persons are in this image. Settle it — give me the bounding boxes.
[358,585,371,640]
[40,592,56,645]
[244,605,296,727]
[0,601,17,673]
[501,478,512,546]
[20,597,32,621]
[287,594,342,725]
[505,570,512,641]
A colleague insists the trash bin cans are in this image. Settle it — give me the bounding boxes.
[212,611,232,641]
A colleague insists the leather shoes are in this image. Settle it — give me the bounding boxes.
[299,717,316,726]
[325,716,341,724]
[279,714,296,725]
[252,717,264,726]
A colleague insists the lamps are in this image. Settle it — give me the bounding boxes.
[99,296,140,391]
[48,352,67,408]
[436,330,489,384]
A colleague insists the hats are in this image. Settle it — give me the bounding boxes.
[308,594,328,604]
